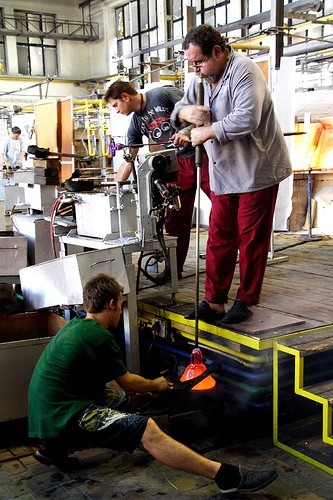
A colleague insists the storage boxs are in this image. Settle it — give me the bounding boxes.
[18,245,131,311]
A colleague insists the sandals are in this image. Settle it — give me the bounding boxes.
[34,448,80,474]
[219,463,279,493]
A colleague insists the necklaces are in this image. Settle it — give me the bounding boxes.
[135,92,144,118]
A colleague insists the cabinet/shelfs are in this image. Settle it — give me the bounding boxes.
[34,99,115,182]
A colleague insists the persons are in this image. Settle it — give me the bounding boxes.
[170,23,293,325]
[103,80,210,283]
[3,126,25,171]
[28,274,278,497]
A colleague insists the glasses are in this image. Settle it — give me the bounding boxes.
[188,50,211,69]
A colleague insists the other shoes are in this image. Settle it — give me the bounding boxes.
[221,300,253,324]
[156,267,182,280]
[184,300,227,321]
